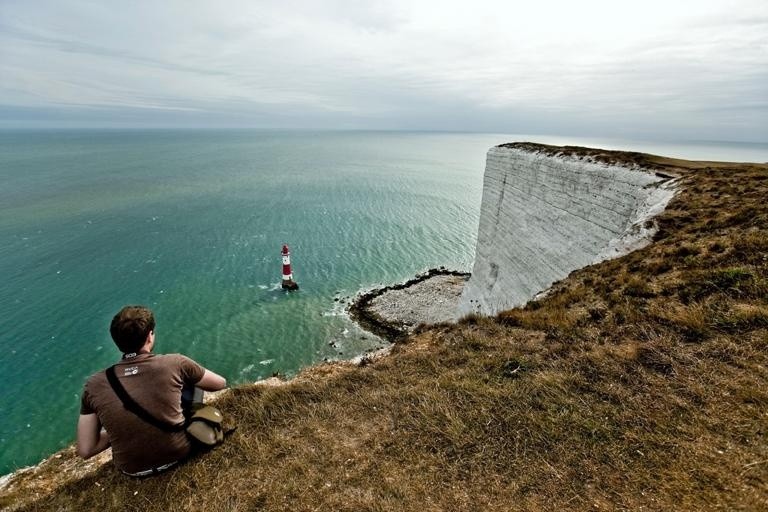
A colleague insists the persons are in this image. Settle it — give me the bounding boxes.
[77,305,228,479]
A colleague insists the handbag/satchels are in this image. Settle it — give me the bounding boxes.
[184,403,224,450]
